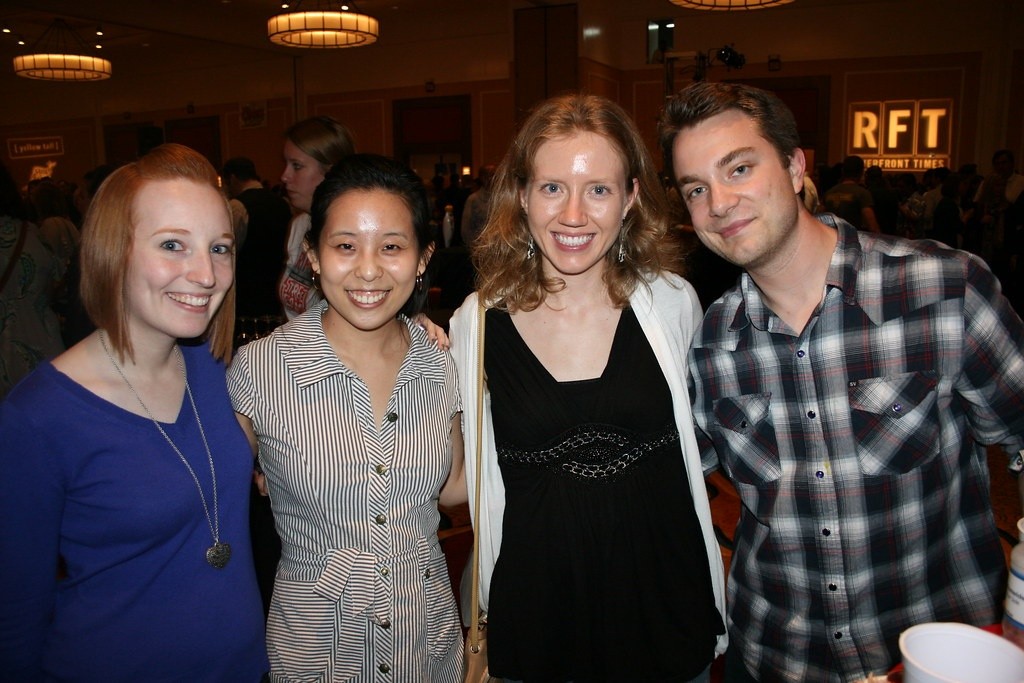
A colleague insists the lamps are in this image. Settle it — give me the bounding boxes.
[707,47,746,69]
[267,0,380,50]
[13,18,112,81]
[667,0,794,11]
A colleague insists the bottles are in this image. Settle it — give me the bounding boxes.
[1001,518,1024,647]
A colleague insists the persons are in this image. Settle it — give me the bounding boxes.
[0,80,1024,683]
[226,152,468,683]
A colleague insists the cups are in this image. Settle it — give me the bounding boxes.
[899,622,1024,683]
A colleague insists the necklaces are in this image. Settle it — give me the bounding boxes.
[97,326,232,569]
